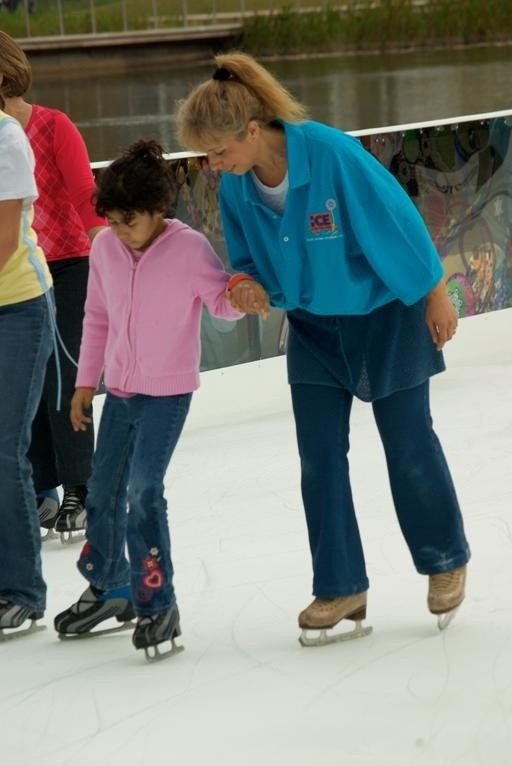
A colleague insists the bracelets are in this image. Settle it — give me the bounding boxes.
[227,271,257,291]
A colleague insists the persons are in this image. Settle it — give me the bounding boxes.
[175,52,473,632]
[0,103,58,630]
[0,28,110,535]
[50,136,276,650]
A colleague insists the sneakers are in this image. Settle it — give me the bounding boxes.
[429,564,467,614]
[299,591,367,630]
[53,487,88,533]
[0,586,179,649]
